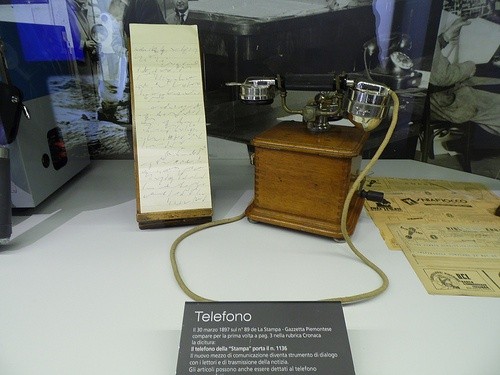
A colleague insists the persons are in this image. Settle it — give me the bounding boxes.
[429,14,500,138]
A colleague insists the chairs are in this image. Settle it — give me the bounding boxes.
[422,90,472,173]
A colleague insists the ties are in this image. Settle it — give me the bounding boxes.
[181,15,184,24]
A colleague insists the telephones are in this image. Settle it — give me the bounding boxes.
[223,71,392,136]
[364,32,422,91]
